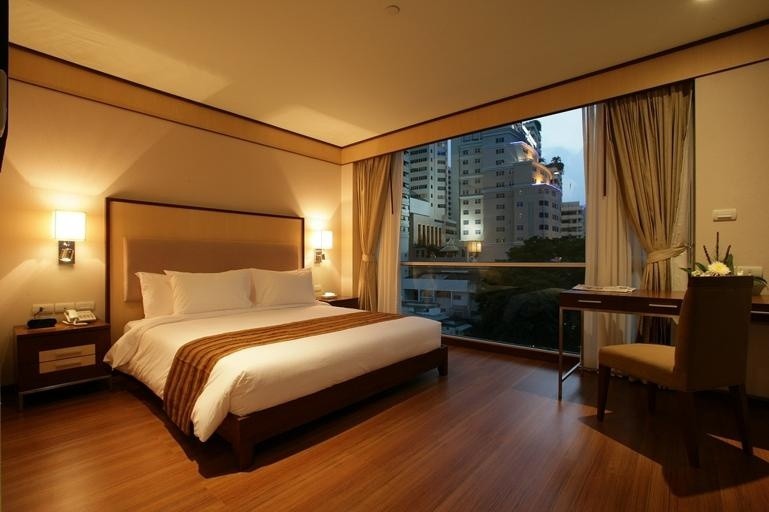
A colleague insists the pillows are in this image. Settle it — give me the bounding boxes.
[250,267,316,307]
[163,268,253,315]
[134,271,174,319]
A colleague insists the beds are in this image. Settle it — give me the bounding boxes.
[102,196,448,473]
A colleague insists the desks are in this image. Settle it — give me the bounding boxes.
[556,285,769,402]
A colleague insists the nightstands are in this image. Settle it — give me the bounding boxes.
[315,295,359,311]
[14,317,112,413]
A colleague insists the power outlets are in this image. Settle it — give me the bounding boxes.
[55,303,74,312]
[33,304,54,314]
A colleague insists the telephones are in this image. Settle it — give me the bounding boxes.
[62,309,96,326]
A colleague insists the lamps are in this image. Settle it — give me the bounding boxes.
[52,210,86,265]
[467,241,481,263]
[311,229,333,263]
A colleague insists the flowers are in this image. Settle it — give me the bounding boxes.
[678,232,768,286]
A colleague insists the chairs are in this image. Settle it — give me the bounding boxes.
[595,275,753,469]
